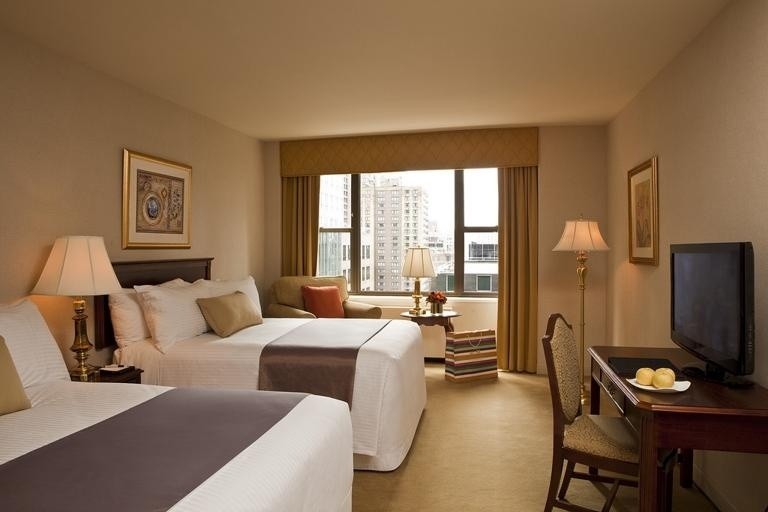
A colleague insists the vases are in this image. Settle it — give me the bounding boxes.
[430,303,443,313]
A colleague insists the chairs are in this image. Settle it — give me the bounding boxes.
[544,314,675,512]
[268,273,382,319]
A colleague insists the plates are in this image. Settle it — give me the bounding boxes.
[626,377,691,394]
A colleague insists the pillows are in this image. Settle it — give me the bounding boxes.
[0,299,71,389]
[206,275,263,316]
[197,291,263,338]
[301,286,345,318]
[0,335,32,415]
[109,278,184,349]
[134,279,217,354]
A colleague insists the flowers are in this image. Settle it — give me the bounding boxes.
[425,291,447,306]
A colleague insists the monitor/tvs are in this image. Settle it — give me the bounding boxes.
[670,241,755,389]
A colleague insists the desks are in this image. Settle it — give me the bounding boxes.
[400,311,462,332]
[588,346,768,512]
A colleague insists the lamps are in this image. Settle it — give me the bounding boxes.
[551,212,611,405]
[29,236,125,382]
[402,245,436,314]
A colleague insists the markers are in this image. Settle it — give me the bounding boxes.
[104,365,124,368]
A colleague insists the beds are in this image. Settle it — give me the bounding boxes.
[93,258,427,471]
[0,382,355,512]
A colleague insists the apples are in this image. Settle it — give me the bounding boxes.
[655,367,675,383]
[652,372,673,388]
[636,368,655,386]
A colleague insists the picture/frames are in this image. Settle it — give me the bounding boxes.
[122,147,194,249]
[628,155,658,266]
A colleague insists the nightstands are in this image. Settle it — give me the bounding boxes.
[76,361,144,385]
